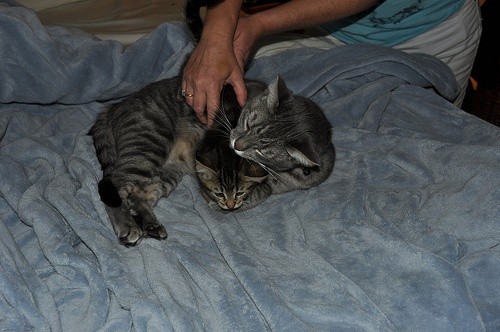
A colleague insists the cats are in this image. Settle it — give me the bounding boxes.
[86,73,336,249]
[177,0,269,217]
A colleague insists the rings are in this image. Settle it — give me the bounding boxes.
[182,89,193,97]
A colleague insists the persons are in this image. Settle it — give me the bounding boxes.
[181,0,483,131]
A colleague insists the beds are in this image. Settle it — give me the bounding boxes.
[1,1,500,332]
